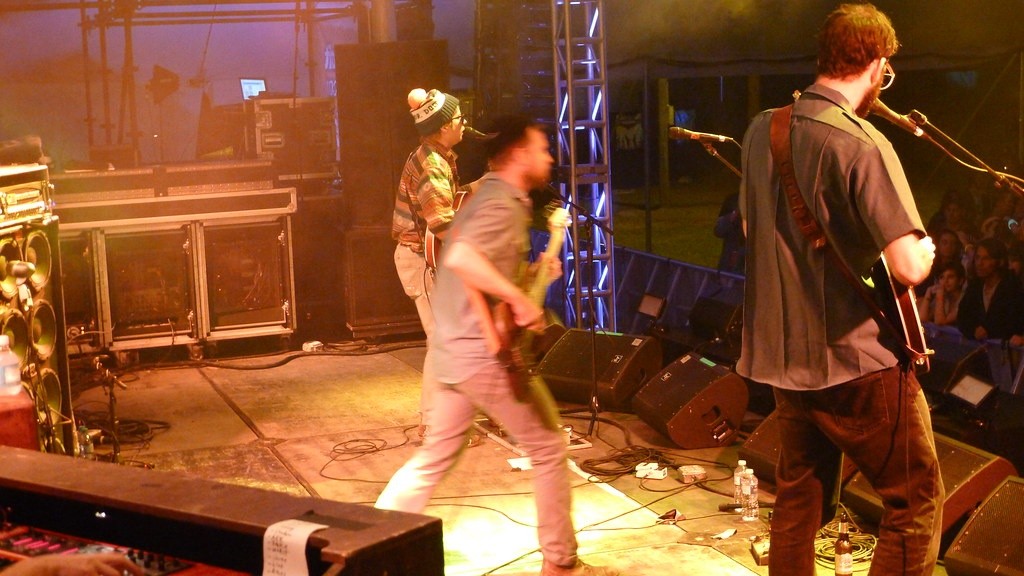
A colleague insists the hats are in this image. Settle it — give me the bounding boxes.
[476,116,554,173]
[407,88,459,136]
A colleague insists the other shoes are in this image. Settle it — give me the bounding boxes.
[540,556,619,576]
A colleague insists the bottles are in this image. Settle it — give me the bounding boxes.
[835,513,852,576]
[734,459,759,521]
[77,425,95,459]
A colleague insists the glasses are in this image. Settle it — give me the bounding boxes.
[452,113,466,124]
[879,63,895,90]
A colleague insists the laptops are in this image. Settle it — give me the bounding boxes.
[240,78,267,101]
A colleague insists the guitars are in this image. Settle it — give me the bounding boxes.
[489,206,574,377]
[423,188,471,270]
[790,87,930,373]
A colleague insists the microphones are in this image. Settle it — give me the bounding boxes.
[463,128,485,140]
[870,97,931,140]
[668,127,734,143]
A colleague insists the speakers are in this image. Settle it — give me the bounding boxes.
[537,326,663,410]
[334,38,453,226]
[844,431,1019,557]
[339,222,422,328]
[948,384,1024,450]
[945,474,1024,576]
[689,297,744,345]
[738,407,861,490]
[524,303,567,368]
[632,352,748,449]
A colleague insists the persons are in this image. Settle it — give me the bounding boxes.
[914,182,1024,429]
[393,89,488,447]
[714,192,745,275]
[739,3,947,576]
[373,127,619,576]
[0,553,143,576]
